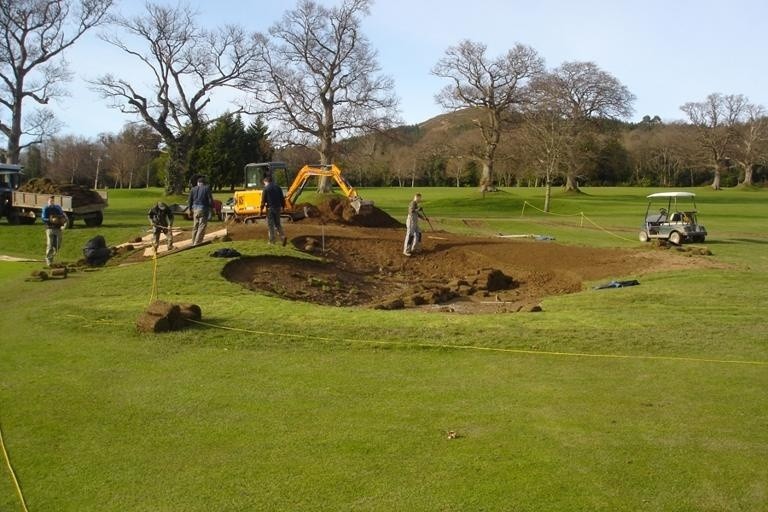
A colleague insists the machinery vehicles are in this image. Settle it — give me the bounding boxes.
[233,161,374,225]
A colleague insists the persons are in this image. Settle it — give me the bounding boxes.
[402,193,429,257]
[188,178,216,248]
[84,234,109,267]
[146,201,175,252]
[41,194,67,266]
[258,175,287,248]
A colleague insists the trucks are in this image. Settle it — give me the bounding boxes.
[0,163,109,227]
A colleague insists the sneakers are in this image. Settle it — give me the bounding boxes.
[281,236,286,247]
[403,250,416,257]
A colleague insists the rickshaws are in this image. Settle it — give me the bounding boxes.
[167,204,225,221]
